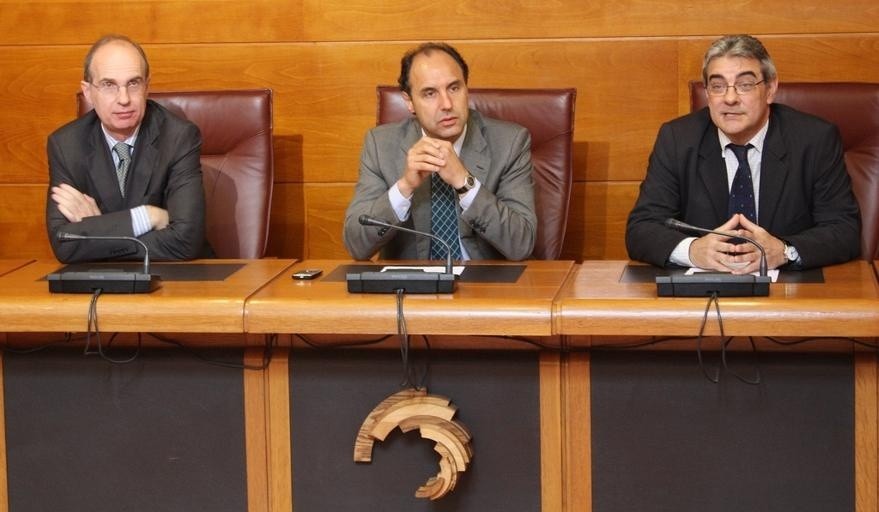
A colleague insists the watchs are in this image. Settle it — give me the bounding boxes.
[785,241,800,263]
[455,172,475,194]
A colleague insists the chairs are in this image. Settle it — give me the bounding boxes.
[689,80,878,262]
[77,89,275,257]
[376,85,576,259]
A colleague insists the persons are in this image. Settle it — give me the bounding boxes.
[45,34,220,262]
[344,41,539,263]
[624,37,864,274]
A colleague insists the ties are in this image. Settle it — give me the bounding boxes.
[725,144,757,257]
[431,173,465,261]
[114,143,132,198]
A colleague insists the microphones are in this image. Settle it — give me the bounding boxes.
[46,232,162,276]
[655,217,770,275]
[345,215,458,275]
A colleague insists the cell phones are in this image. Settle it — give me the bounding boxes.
[291,268,323,280]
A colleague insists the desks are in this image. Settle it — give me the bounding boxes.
[244,259,581,512]
[1,259,298,512]
[561,259,879,511]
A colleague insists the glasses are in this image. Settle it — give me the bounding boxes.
[91,80,143,91]
[706,79,765,97]
